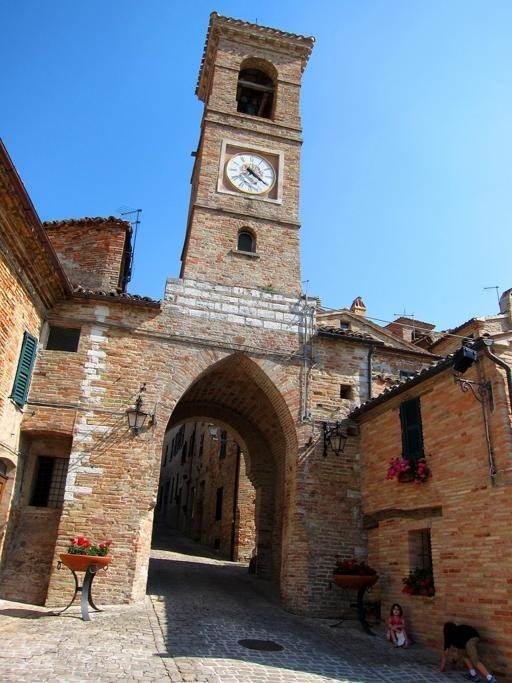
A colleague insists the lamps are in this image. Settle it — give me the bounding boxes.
[126,382,149,432]
[207,423,233,441]
[322,420,348,457]
[447,346,494,413]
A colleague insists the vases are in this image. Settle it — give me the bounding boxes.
[399,477,414,481]
[48,553,112,621]
[329,575,381,636]
[402,586,416,594]
[418,588,428,595]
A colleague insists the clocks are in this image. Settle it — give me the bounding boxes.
[225,151,277,195]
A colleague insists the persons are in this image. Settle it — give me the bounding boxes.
[386,603,410,648]
[435,622,496,683]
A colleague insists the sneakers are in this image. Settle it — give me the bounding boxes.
[464,673,480,682]
[479,675,497,683]
[392,639,410,648]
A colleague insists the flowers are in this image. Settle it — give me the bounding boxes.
[405,449,433,487]
[400,566,416,587]
[68,537,112,557]
[384,456,412,480]
[412,566,435,596]
[333,555,377,576]
